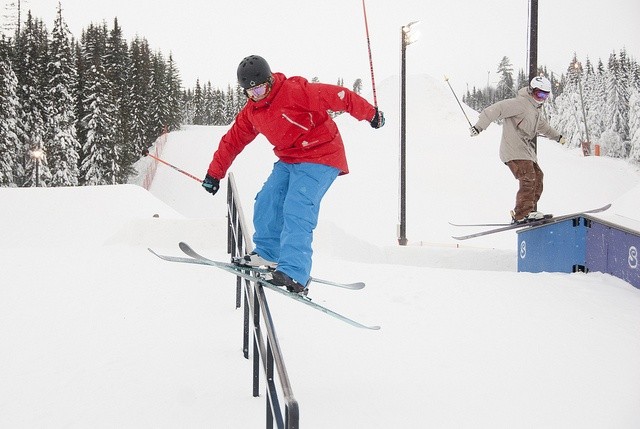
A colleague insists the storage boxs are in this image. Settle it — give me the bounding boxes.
[515,213,639,289]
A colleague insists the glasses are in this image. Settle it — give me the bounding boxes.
[530,87,552,102]
[244,81,271,99]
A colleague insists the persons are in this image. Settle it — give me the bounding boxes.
[469,74,566,224]
[201,53,386,293]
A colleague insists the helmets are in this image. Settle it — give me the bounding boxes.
[529,76,553,106]
[237,55,270,89]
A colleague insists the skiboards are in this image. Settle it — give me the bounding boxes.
[148,242,380,330]
[448,203,611,240]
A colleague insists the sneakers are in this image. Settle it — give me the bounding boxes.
[246,252,279,269]
[268,269,305,294]
[510,210,543,224]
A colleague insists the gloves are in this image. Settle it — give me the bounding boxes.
[370,110,384,129]
[470,125,481,136]
[201,174,219,195]
[559,135,568,146]
